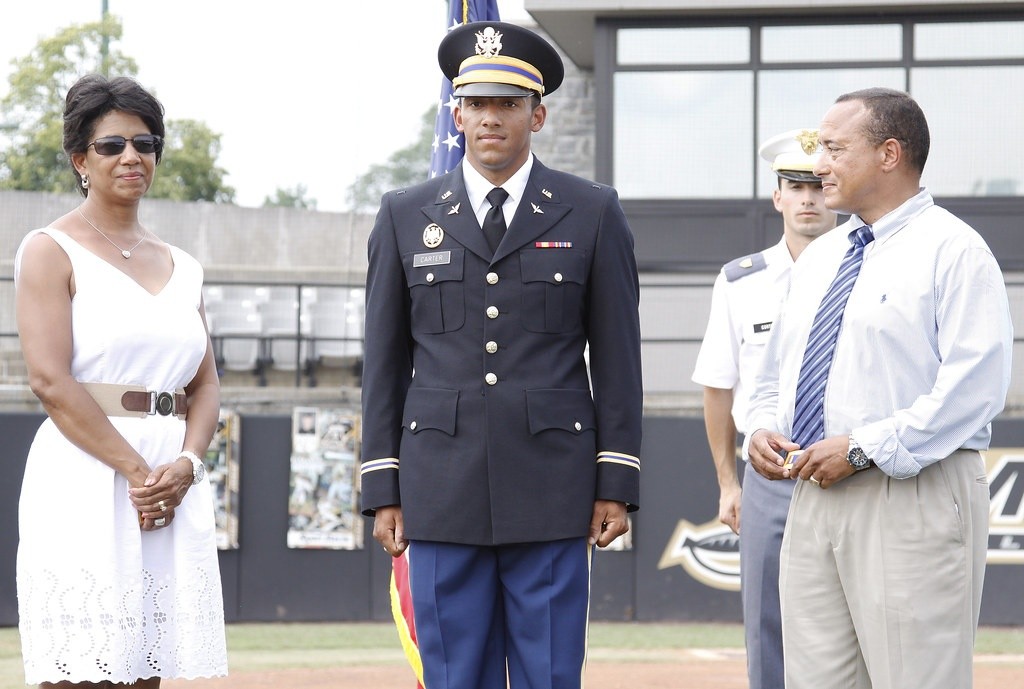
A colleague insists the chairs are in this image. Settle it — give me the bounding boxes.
[203,298,365,387]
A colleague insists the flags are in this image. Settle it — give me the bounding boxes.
[389,0,498,689]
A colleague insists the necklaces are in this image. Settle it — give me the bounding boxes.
[79,208,147,258]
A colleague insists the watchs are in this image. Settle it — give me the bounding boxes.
[176,451,206,485]
[848,434,870,470]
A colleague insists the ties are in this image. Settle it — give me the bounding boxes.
[481,187,509,257]
[792,225,874,453]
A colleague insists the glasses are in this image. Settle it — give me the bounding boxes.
[82,134,163,155]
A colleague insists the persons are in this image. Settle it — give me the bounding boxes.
[745,90,1012,689]
[13,76,230,689]
[360,20,641,689]
[299,415,315,433]
[689,129,836,689]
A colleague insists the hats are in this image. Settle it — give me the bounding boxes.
[439,22,563,106]
[758,129,824,182]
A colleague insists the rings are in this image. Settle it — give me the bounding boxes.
[155,517,165,525]
[809,476,821,486]
[158,501,167,511]
